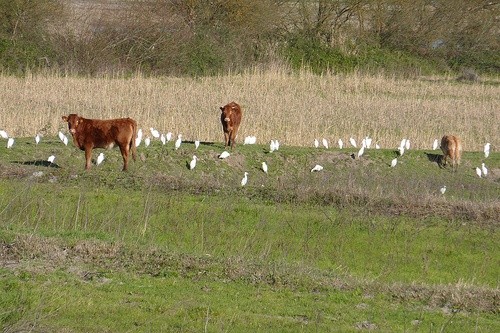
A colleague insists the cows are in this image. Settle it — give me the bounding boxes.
[218,101,243,149]
[61,112,138,173]
[440,133,461,174]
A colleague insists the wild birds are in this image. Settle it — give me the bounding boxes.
[0,129,15,149]
[174,134,182,151]
[34,133,40,145]
[481,162,487,177]
[243,135,257,145]
[94,152,105,166]
[189,155,198,170]
[166,132,173,142]
[314,138,319,148]
[144,136,151,147]
[218,150,230,160]
[58,130,68,146]
[150,127,160,140]
[310,163,324,173]
[135,129,142,147]
[322,138,328,149]
[475,167,482,178]
[160,134,166,145]
[482,142,491,159]
[349,136,380,157]
[46,154,55,163]
[193,140,199,149]
[268,138,280,153]
[398,138,411,156]
[240,171,249,187]
[439,185,447,194]
[337,139,343,150]
[432,138,438,151]
[260,161,268,174]
[390,157,398,167]
